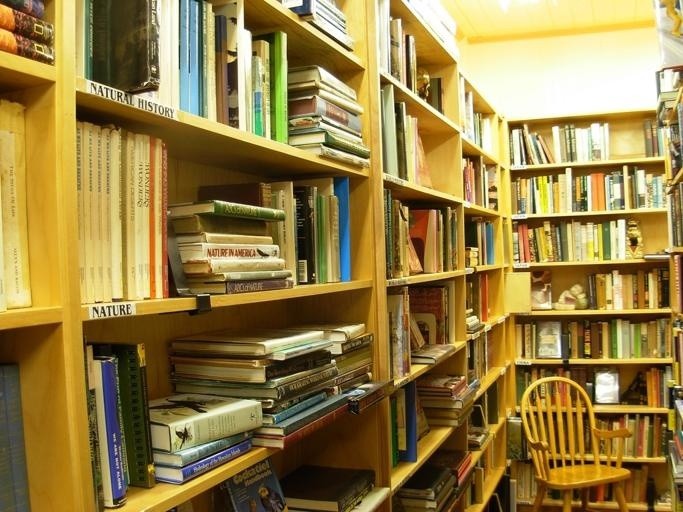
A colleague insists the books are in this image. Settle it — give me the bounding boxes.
[510,120,675,502]
[0,0,375,511]
[379,1,511,511]
[656,1,682,511]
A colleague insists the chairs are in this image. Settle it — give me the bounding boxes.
[521,376,632,512]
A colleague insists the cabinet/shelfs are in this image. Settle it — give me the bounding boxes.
[509,0,683,512]
[0,0,508,512]
